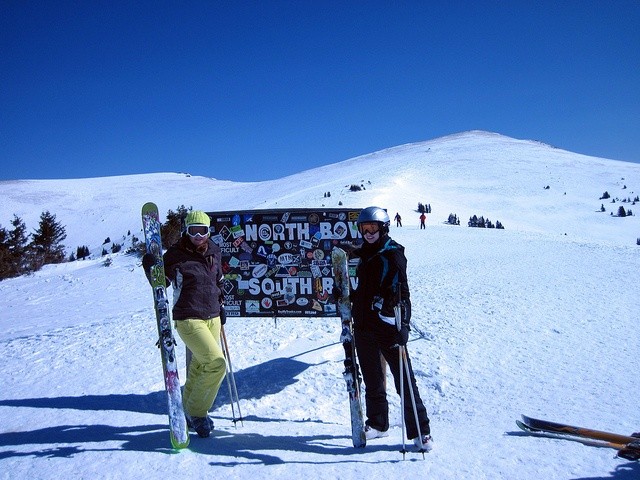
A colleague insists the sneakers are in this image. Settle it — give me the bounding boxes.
[365,425,389,440]
[415,434,433,451]
[186,411,214,438]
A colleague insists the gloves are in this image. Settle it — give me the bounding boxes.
[394,326,409,348]
[142,252,157,271]
[220,306,226,325]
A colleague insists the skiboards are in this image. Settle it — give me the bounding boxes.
[515,414,640,454]
[331,247,366,447]
[142,201,191,449]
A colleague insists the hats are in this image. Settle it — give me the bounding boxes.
[185,211,211,227]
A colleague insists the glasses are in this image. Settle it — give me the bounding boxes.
[357,223,380,235]
[187,225,210,237]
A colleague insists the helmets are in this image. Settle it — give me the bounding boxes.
[358,207,391,235]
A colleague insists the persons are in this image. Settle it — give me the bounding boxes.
[394,213,403,227]
[142,211,226,437]
[332,205,434,453]
[420,212,426,230]
